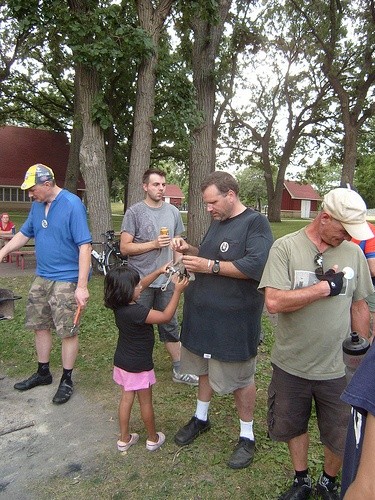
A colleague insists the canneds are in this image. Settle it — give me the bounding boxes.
[160,227,169,235]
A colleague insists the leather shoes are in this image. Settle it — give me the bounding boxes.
[227,435,255,469]
[174,413,211,447]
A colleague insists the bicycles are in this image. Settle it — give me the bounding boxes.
[90,229,128,276]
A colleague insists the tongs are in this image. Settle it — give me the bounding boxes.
[70,304,82,336]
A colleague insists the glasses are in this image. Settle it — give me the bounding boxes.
[314,252,324,281]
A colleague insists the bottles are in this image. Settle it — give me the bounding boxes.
[341,331,370,384]
[91,250,100,259]
[11,227,15,235]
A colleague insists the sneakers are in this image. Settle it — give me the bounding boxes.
[145,431,166,451]
[13,371,53,390]
[316,474,342,500]
[280,477,312,500]
[116,432,140,452]
[52,379,74,405]
[171,365,200,386]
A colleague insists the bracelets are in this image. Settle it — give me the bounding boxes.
[208,259,211,273]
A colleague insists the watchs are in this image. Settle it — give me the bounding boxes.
[212,260,220,276]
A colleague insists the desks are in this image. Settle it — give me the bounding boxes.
[0,235,19,268]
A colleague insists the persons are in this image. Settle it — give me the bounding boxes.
[0,213,15,262]
[103,261,190,451]
[169,172,274,469]
[120,168,199,385]
[0,164,92,404]
[257,188,375,500]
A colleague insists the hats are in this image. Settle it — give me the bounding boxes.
[19,162,55,191]
[320,187,375,241]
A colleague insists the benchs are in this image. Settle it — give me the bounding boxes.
[2,244,35,262]
[9,251,35,271]
